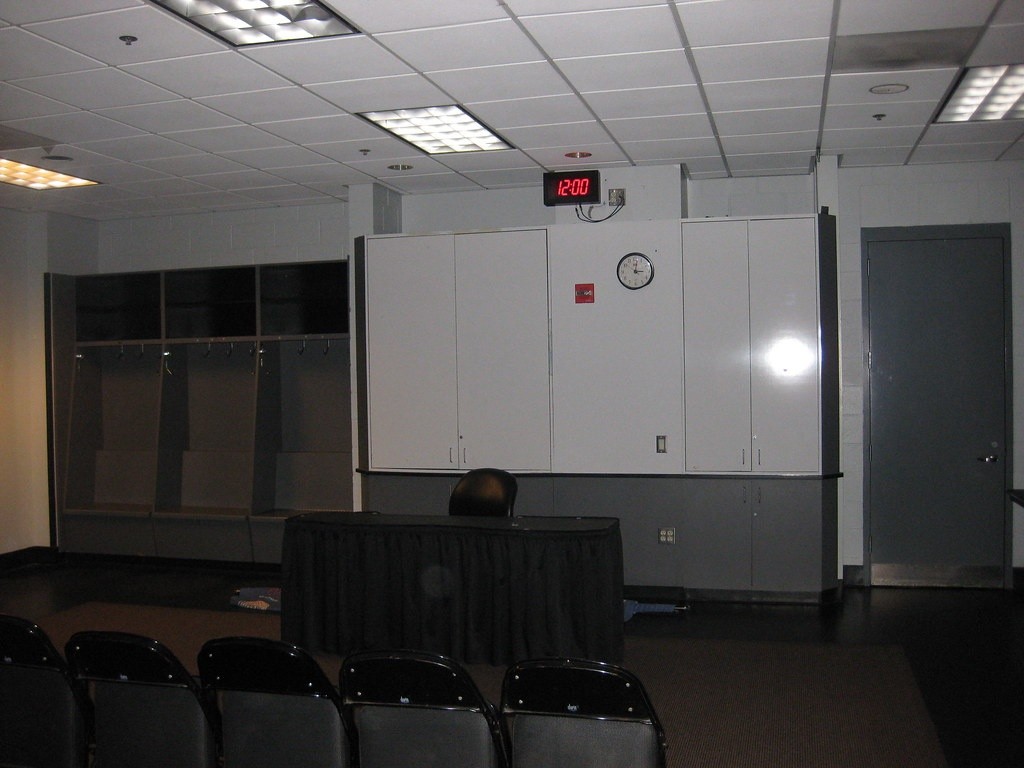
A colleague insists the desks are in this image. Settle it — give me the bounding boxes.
[281,512,622,667]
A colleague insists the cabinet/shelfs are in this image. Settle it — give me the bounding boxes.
[360,473,553,519]
[365,227,552,473]
[683,479,838,601]
[44,260,350,557]
[682,213,822,476]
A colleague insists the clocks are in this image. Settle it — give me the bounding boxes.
[617,252,654,290]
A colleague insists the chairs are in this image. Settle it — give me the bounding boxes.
[448,469,518,517]
[0,614,671,768]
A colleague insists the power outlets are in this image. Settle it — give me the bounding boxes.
[657,528,676,546]
[608,189,625,206]
